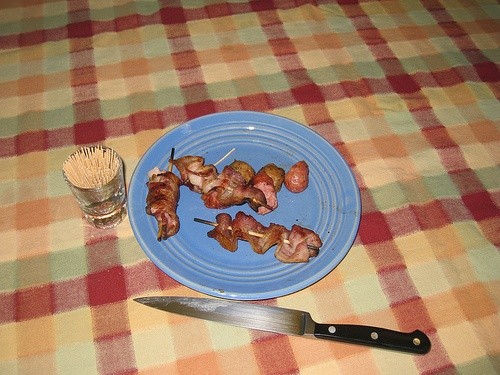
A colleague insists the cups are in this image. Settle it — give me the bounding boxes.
[62,145,128,230]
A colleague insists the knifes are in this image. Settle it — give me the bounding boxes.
[133,297,432,357]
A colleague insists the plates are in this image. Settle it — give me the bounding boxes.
[128,110,361,301]
